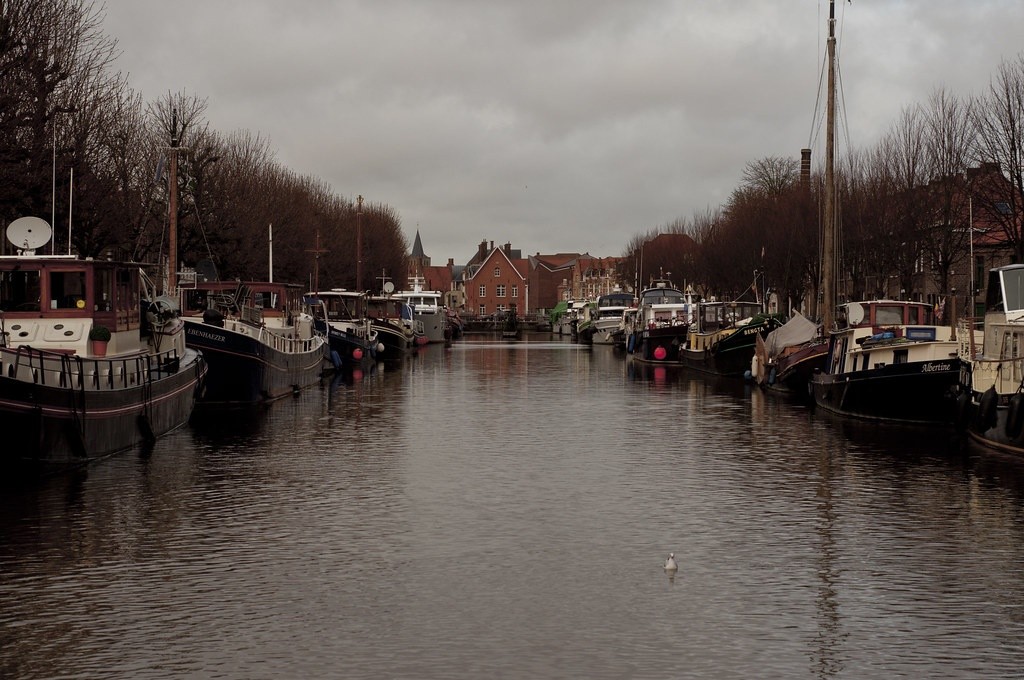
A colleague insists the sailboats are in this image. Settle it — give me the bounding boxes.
[355,195,425,357]
[139,109,324,405]
[753,0,842,393]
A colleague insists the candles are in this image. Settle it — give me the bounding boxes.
[90,326,111,355]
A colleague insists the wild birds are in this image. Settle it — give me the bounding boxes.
[663,552,679,571]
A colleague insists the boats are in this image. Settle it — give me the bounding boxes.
[626,287,697,363]
[305,230,379,371]
[0,122,209,461]
[446,310,463,340]
[610,308,638,348]
[593,293,640,332]
[808,300,958,424]
[395,270,446,342]
[956,198,1024,455]
[679,301,785,376]
[577,304,598,340]
